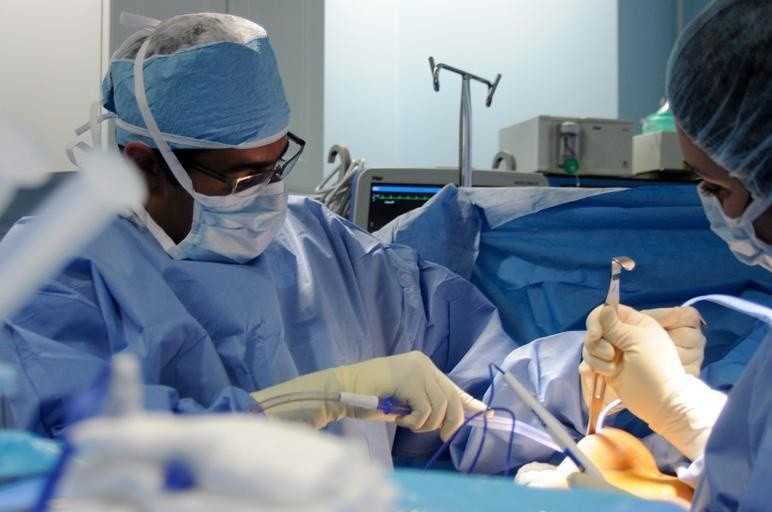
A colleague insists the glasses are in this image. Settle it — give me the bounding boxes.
[153,133,306,198]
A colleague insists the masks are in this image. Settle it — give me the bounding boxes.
[122,129,289,265]
[695,177,772,274]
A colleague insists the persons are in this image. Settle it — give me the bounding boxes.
[0,10,710,474]
[514,0,771,511]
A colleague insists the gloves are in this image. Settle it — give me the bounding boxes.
[327,348,496,443]
[582,298,730,464]
[566,473,638,498]
[577,302,708,417]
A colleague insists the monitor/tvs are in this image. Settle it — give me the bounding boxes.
[348,167,549,233]
